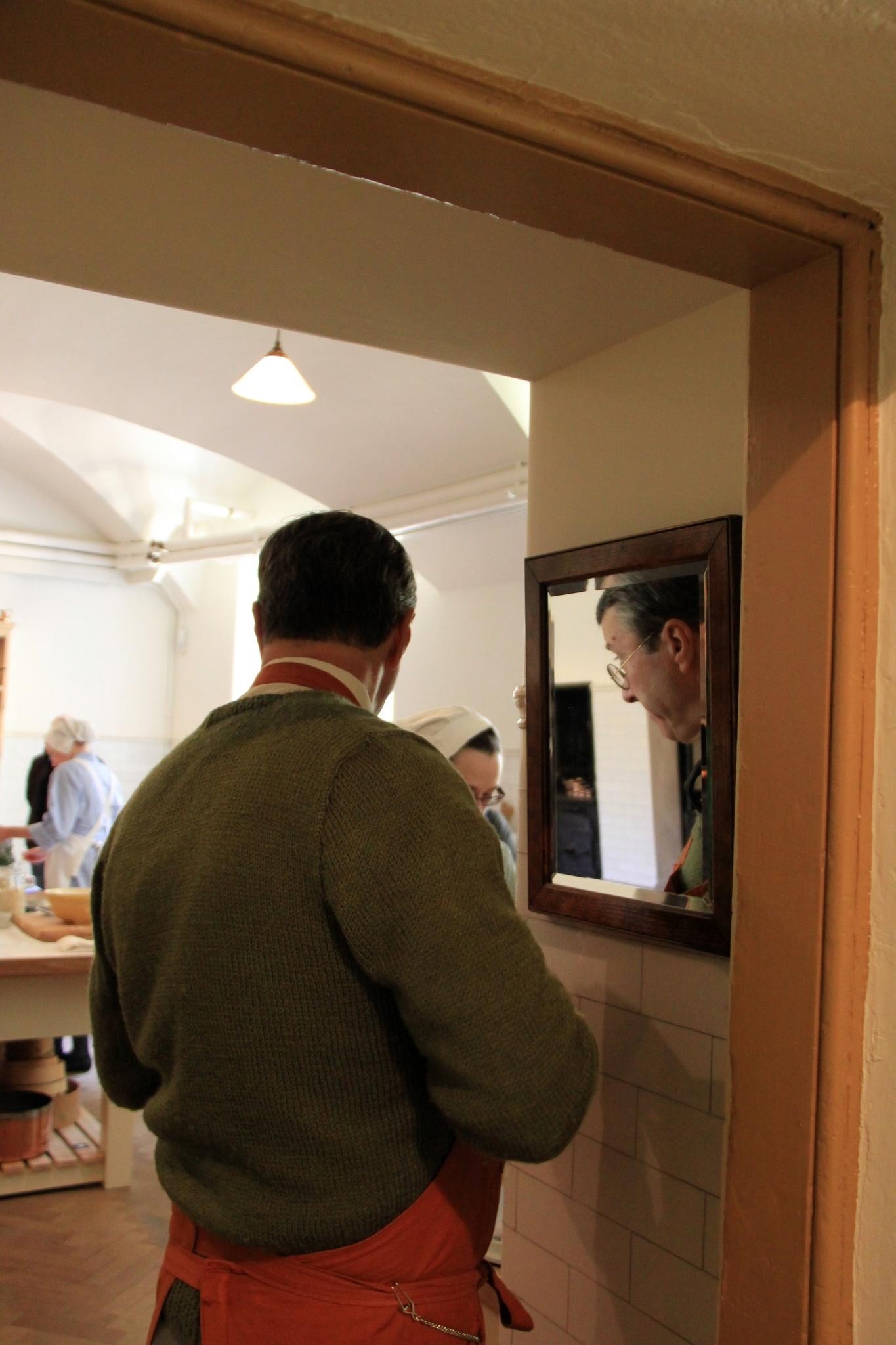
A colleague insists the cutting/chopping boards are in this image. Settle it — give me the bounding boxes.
[10,911,93,942]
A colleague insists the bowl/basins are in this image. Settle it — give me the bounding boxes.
[44,887,91,926]
[0,1078,80,1164]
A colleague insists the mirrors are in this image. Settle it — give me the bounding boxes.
[523,515,742,959]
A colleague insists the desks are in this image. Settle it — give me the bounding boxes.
[0,925,135,1198]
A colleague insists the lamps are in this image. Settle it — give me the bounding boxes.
[231,329,317,405]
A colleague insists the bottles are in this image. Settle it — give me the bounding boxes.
[23,874,42,912]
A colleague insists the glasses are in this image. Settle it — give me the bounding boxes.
[607,634,653,690]
[476,787,505,807]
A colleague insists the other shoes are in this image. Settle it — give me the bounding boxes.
[61,1048,93,1075]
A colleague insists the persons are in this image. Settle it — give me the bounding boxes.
[593,575,705,904]
[88,514,596,1343]
[0,716,122,895]
[397,703,520,907]
